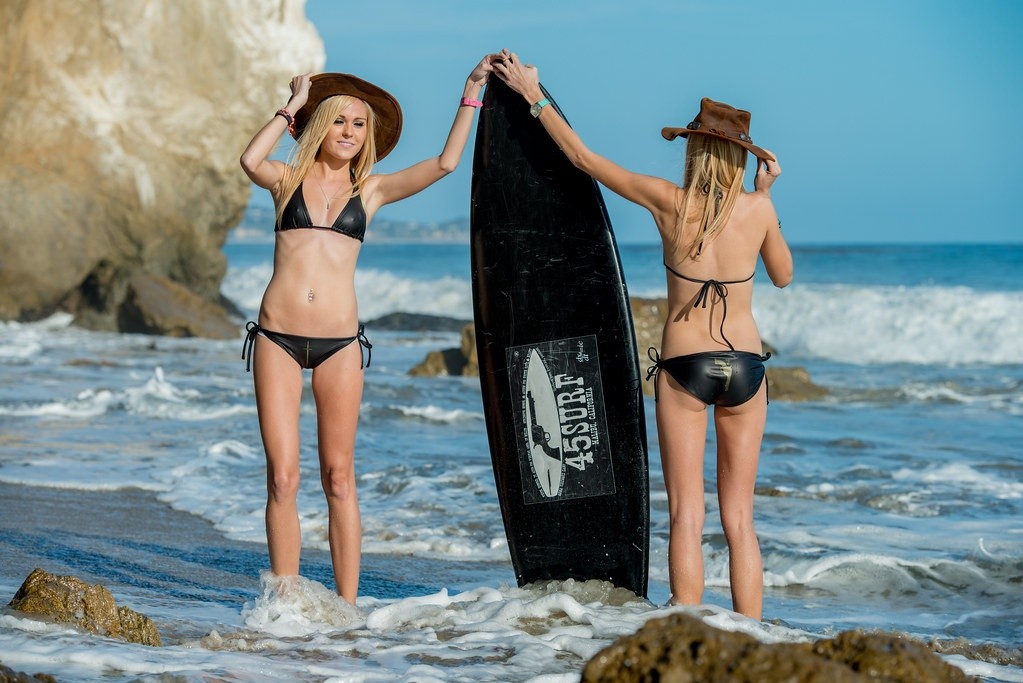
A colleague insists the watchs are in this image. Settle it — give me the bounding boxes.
[530,98,550,118]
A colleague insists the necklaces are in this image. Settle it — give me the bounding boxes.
[313,165,345,209]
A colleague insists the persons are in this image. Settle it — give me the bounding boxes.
[240,56,500,609]
[493,50,791,622]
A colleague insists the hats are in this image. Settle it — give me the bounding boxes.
[662,97,776,162]
[287,72,403,164]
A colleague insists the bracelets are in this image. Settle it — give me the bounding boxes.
[461,97,483,107]
[778,219,781,229]
[275,109,295,134]
